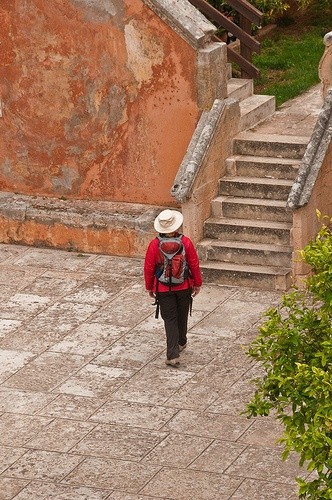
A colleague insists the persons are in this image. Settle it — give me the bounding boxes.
[144,209,203,366]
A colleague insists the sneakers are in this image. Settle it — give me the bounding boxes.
[178,343,187,353]
[165,357,180,366]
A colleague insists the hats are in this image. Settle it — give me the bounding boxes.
[153,208,184,233]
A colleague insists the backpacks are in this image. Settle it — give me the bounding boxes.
[155,234,190,286]
